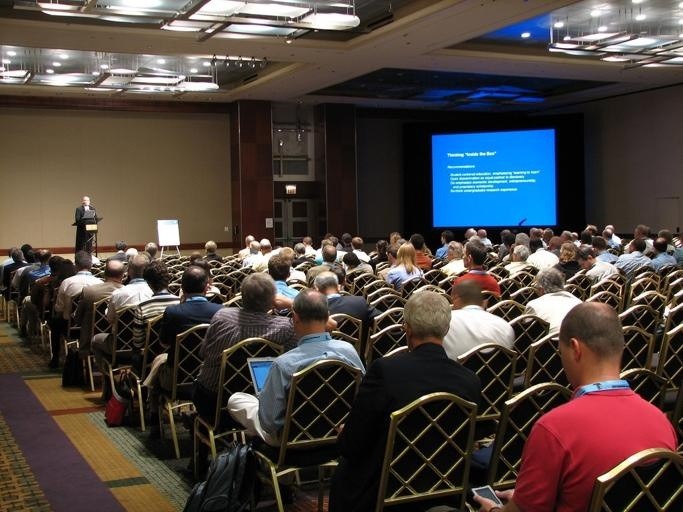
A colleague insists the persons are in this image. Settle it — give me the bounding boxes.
[0,222,682,511]
[72,195,100,255]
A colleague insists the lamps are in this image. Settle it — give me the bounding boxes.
[545,1,683,73]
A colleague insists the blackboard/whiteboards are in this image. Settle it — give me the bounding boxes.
[157,219,180,246]
